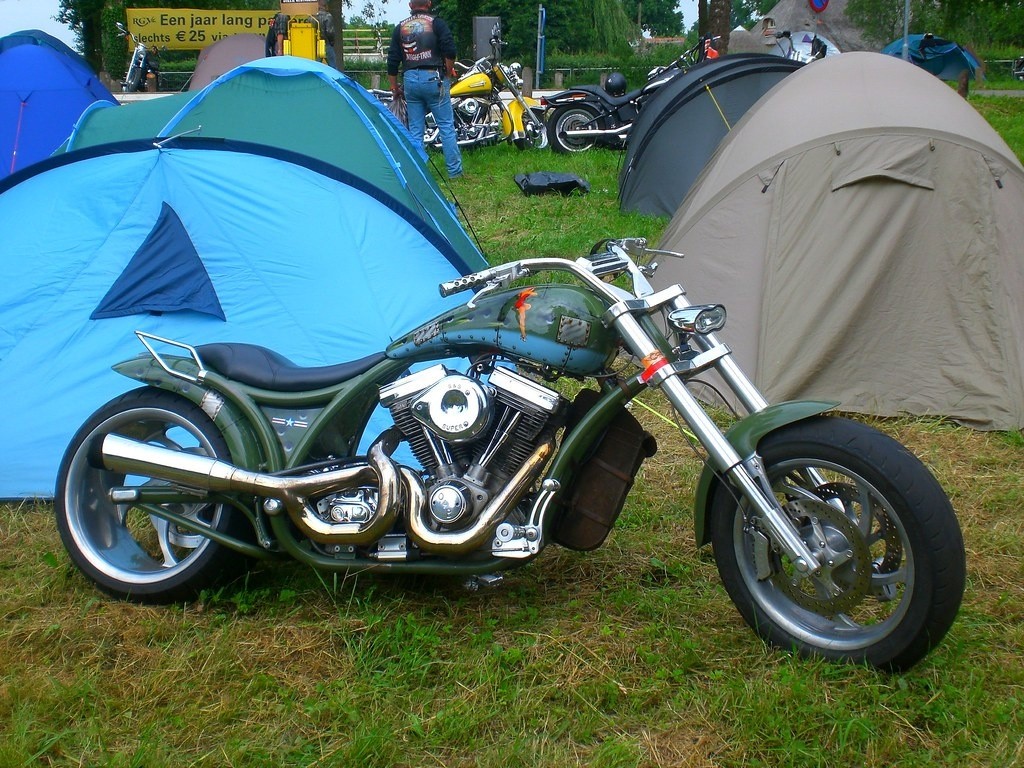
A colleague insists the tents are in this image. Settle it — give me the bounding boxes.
[0,28,517,506]
[624,31,1023,440]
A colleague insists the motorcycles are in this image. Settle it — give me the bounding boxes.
[360,20,827,157]
[114,22,167,93]
[48,233,967,679]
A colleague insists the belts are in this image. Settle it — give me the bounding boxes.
[412,66,439,69]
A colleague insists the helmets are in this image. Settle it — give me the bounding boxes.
[605,72,627,97]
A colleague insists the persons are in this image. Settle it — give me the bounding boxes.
[313,0,337,69]
[388,1,470,182]
[273,12,291,57]
